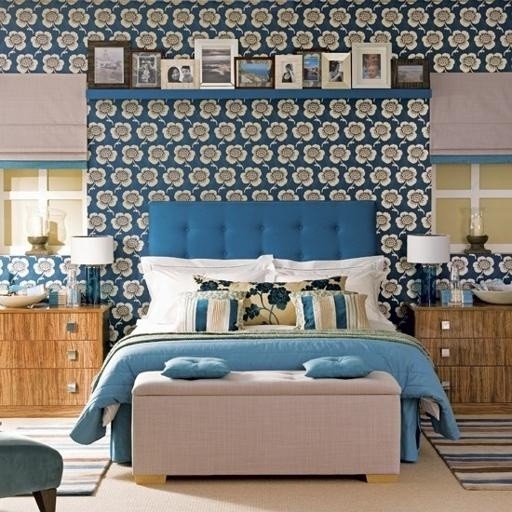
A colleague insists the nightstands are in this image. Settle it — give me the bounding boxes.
[409,304,512,413]
[0,304,109,419]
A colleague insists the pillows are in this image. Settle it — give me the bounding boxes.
[128,254,398,333]
[159,355,230,378]
[300,355,372,379]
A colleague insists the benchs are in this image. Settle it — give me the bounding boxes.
[130,369,402,484]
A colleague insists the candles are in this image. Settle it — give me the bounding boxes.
[472,215,483,236]
[31,215,43,237]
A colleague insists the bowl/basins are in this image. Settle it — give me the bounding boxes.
[470,287,512,304]
[0,294,43,307]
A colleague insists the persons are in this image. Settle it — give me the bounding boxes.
[329,62,342,83]
[364,53,380,81]
[166,66,193,84]
[283,62,296,83]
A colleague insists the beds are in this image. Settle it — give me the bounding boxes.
[109,200,420,468]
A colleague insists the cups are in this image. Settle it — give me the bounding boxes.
[48,284,68,306]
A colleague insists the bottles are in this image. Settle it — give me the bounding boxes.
[66,269,81,306]
[450,264,461,304]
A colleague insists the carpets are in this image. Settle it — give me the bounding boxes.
[0,419,112,496]
[417,413,512,490]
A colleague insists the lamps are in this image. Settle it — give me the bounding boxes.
[69,234,115,305]
[406,233,450,306]
